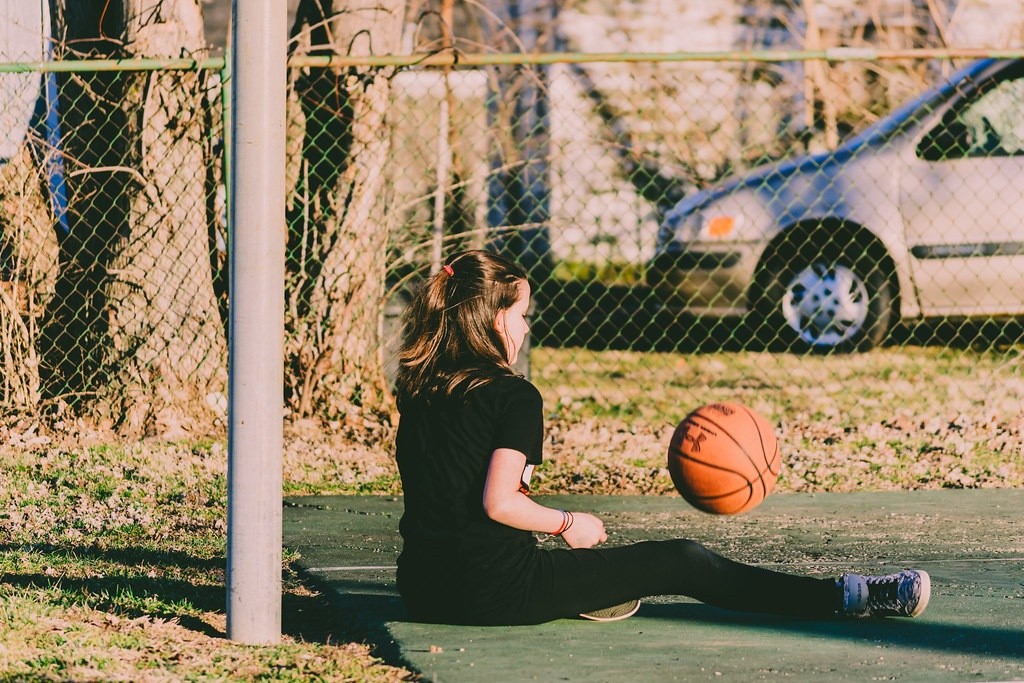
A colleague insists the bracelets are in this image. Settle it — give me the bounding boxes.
[551,509,574,536]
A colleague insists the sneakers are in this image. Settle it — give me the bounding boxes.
[580,600,641,622]
[840,569,930,618]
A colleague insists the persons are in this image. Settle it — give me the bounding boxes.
[396,250,932,627]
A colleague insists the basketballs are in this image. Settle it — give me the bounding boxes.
[666,402,786,518]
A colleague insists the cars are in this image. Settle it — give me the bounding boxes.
[644,59,1024,354]
